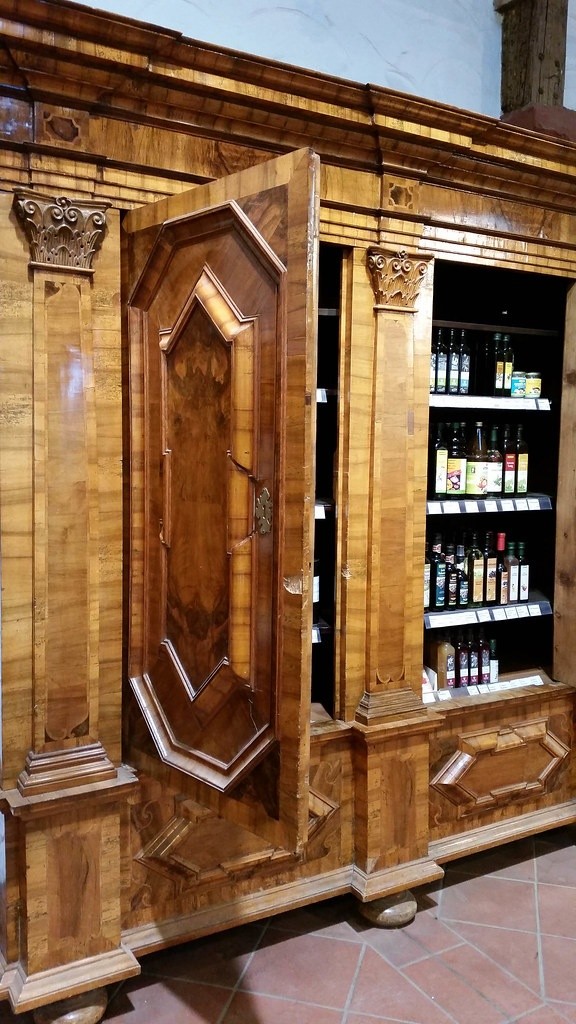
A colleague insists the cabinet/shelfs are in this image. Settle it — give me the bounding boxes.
[0,0,576,1003]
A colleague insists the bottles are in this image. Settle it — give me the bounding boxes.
[425,418,530,501]
[421,631,502,692]
[422,528,530,611]
[430,327,542,399]
